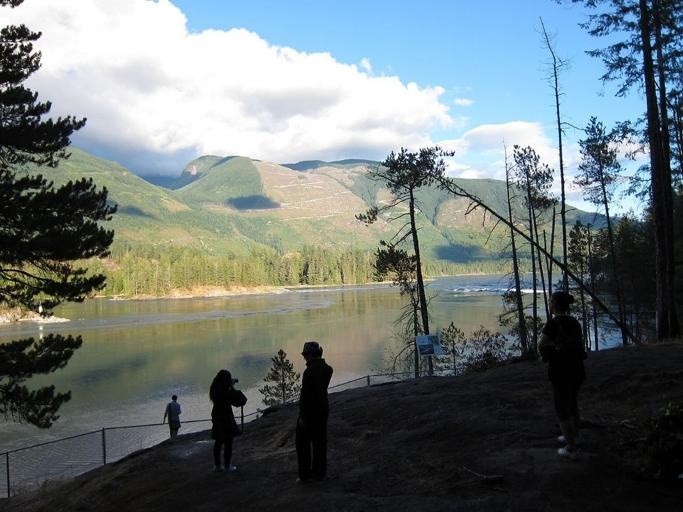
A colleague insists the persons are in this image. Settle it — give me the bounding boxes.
[207,369,247,473]
[536,290,587,461]
[285,341,334,485]
[161,394,182,438]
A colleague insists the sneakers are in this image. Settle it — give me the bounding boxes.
[295,477,325,487]
[213,464,236,472]
[557,434,584,460]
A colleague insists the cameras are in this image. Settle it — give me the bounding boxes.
[231,377,239,385]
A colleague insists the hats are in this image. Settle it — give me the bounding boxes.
[301,342,323,357]
